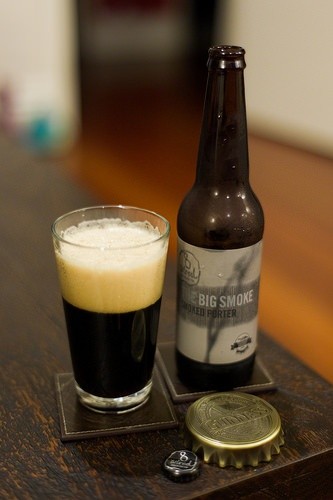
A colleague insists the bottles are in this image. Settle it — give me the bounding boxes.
[175,45,263,392]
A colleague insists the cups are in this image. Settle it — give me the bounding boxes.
[53,206,170,414]
